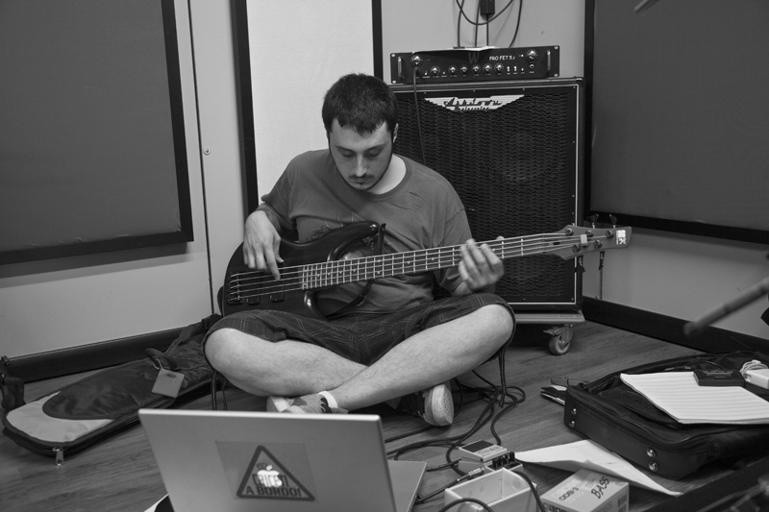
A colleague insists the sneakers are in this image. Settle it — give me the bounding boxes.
[394,381,454,426]
[266,394,331,414]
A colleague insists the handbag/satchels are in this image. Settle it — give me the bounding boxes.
[564,351,769,480]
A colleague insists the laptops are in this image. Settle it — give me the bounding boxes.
[138,408,428,510]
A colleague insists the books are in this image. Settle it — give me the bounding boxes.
[617,370,769,429]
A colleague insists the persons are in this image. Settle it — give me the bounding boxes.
[197,71,520,430]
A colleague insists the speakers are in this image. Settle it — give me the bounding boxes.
[392,81,586,311]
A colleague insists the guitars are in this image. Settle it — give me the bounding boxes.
[222,223,632,319]
